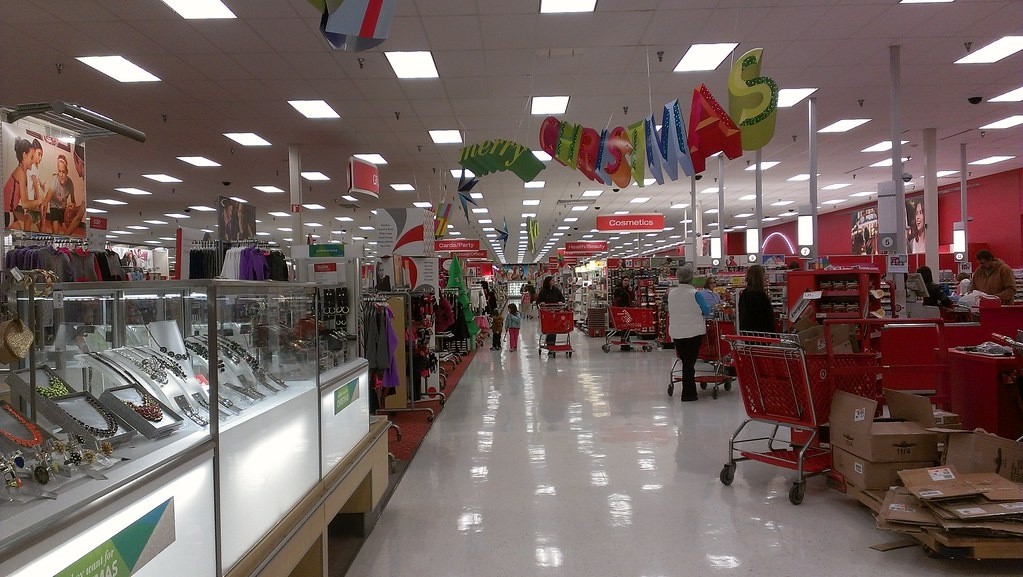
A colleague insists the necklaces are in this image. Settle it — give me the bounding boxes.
[0,324,258,447]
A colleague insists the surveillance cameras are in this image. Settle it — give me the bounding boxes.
[574,228,578,230]
[613,189,620,192]
[789,210,794,212]
[595,207,600,210]
[902,173,913,182]
[969,97,982,104]
[568,234,571,236]
[695,175,702,180]
[184,210,190,212]
[222,182,231,186]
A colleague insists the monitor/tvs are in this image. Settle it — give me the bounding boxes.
[908,273,929,297]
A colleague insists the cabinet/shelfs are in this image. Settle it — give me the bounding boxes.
[567,254,1022,436]
[452,282,510,370]
[365,292,459,424]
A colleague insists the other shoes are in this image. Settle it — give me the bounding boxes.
[510,349,513,351]
[681,395,699,401]
[496,347,500,350]
[514,348,517,350]
[490,346,495,349]
[621,347,633,351]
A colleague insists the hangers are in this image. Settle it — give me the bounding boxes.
[190,238,282,251]
[439,287,459,296]
[363,295,388,306]
[19,235,111,250]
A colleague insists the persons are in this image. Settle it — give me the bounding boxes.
[219,200,253,241]
[957,251,1017,305]
[481,303,520,352]
[124,250,143,267]
[726,256,736,266]
[536,276,564,355]
[376,262,391,292]
[614,277,635,350]
[701,277,721,306]
[765,255,782,265]
[906,199,925,253]
[917,266,954,308]
[738,265,775,346]
[668,267,711,401]
[4,137,86,235]
[853,227,872,254]
[520,281,535,319]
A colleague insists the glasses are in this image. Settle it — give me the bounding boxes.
[709,281,717,284]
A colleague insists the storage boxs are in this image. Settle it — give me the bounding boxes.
[773,316,854,355]
[829,387,1023,495]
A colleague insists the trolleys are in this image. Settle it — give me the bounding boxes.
[720,330,883,506]
[667,314,737,399]
[601,301,656,353]
[947,304,980,322]
[538,303,575,358]
